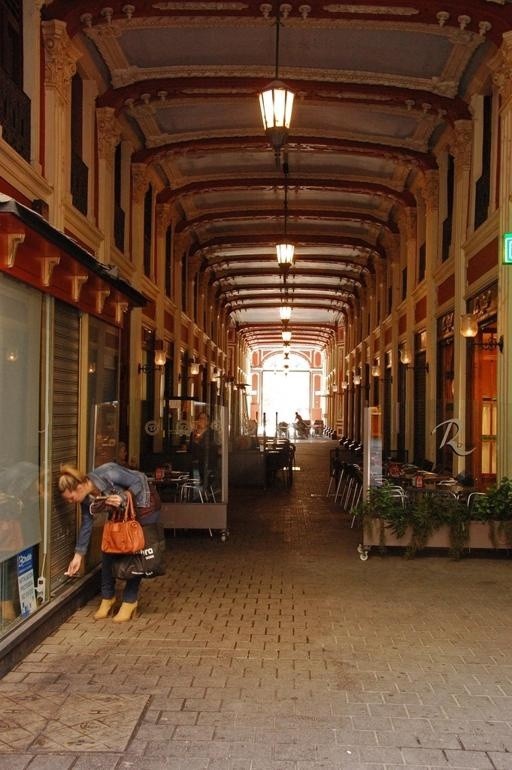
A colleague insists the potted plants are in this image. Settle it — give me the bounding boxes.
[352,478,512,561]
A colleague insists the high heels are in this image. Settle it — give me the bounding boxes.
[111,600,138,622]
[94,595,117,619]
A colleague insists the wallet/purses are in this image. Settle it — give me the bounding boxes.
[90,495,115,513]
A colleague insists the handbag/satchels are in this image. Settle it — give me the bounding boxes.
[111,489,165,580]
[101,490,145,555]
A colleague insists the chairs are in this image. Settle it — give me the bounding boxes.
[174,467,221,537]
[327,448,489,529]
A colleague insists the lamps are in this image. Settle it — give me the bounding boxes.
[257,1,297,376]
[332,308,504,397]
[139,347,237,393]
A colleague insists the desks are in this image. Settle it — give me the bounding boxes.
[145,471,190,496]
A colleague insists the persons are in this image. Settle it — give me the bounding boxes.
[186,411,219,483]
[294,411,303,426]
[56,460,162,624]
